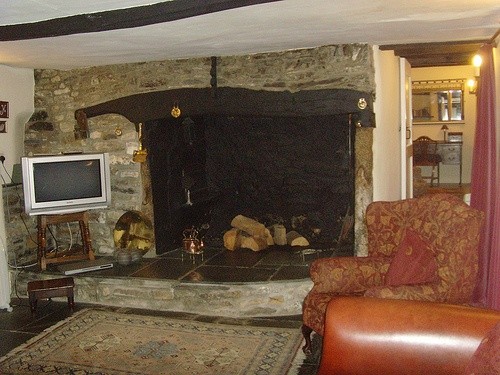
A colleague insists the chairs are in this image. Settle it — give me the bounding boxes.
[413,136,442,188]
[301,189,500,375]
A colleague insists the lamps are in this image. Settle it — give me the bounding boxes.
[441,124,449,140]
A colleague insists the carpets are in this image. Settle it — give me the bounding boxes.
[0,306,315,375]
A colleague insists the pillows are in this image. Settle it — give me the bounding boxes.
[384,230,440,286]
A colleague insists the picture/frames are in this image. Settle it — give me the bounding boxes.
[0,100,9,118]
[0,121,7,133]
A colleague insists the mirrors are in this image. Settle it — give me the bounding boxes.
[412,88,464,124]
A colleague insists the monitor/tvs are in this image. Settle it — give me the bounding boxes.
[21,152,111,215]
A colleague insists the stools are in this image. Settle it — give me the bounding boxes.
[27,277,75,319]
[37,211,96,271]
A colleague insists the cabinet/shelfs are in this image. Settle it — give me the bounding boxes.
[436,140,463,184]
